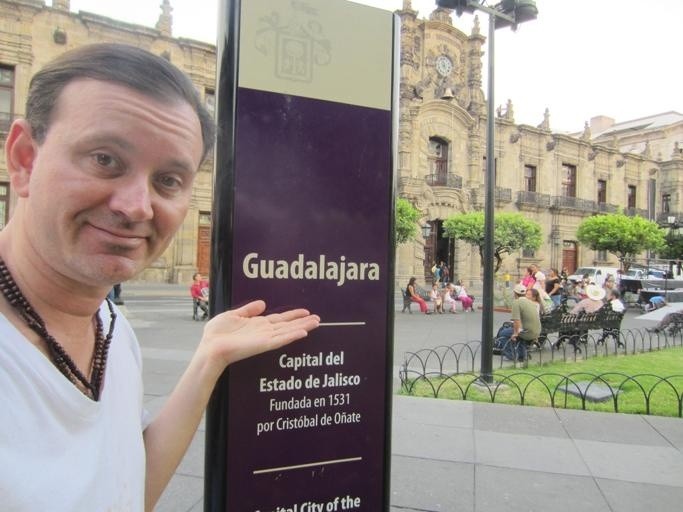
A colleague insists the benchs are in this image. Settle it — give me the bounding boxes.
[400,279,475,314]
[667,312,683,337]
[191,286,210,323]
[491,297,626,359]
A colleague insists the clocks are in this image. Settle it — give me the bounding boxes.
[435,54,454,78]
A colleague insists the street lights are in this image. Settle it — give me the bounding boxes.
[435,0,538,383]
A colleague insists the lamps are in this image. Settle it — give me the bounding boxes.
[438,86,454,100]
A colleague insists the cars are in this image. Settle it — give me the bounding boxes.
[568,266,665,287]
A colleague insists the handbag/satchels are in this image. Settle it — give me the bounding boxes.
[497,322,513,337]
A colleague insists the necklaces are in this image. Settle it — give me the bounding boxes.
[0,259,116,404]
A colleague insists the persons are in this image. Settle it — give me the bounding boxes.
[191,272,209,320]
[432,259,473,314]
[0,44,322,511]
[406,277,431,314]
[493,264,625,359]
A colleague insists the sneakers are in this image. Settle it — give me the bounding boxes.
[551,342,558,350]
[646,327,659,332]
[425,307,472,314]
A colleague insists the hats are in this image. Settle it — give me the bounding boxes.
[514,284,526,295]
[586,285,606,300]
